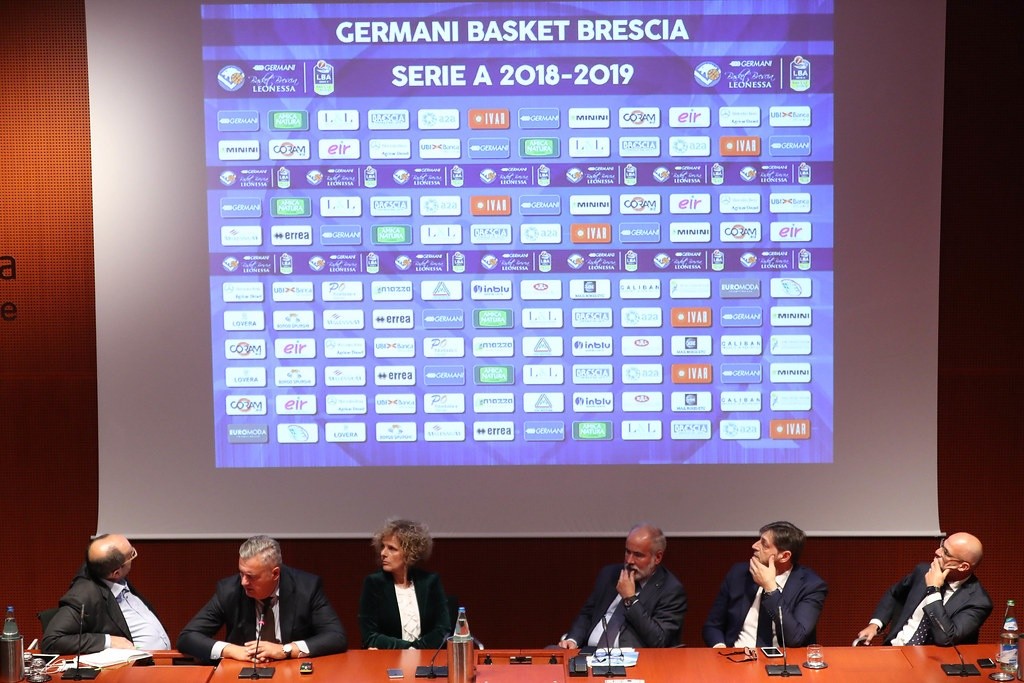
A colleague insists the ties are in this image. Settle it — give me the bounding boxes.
[260,597,275,643]
[756,586,773,647]
[904,581,950,645]
[597,581,642,648]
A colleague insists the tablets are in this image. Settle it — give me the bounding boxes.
[33,654,60,666]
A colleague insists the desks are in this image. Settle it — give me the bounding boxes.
[0,646,1023,683]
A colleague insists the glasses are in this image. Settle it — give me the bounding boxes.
[717,647,757,663]
[940,538,970,564]
[593,646,624,663]
[112,547,138,577]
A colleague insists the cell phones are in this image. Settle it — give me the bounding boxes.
[300,662,313,673]
[387,669,404,678]
[579,646,598,655]
[978,658,994,668]
[761,647,783,658]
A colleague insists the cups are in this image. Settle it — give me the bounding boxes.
[24,652,32,673]
[30,658,46,680]
[807,644,823,668]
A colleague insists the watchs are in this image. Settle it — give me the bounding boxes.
[926,586,940,594]
[283,644,292,660]
[624,595,637,607]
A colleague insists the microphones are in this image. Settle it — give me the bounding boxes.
[932,616,981,675]
[592,615,626,677]
[765,603,803,676]
[238,614,275,679]
[416,633,451,678]
[61,603,101,680]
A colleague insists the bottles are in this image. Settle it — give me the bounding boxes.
[2,607,20,639]
[451,607,470,642]
[999,600,1018,672]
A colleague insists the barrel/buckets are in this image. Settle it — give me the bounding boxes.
[1017,633,1024,681]
[0,635,25,683]
[447,637,475,683]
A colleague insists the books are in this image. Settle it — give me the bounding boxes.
[76,647,152,667]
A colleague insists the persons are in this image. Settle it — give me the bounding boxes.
[41,534,171,656]
[701,521,827,649]
[858,532,994,646]
[558,523,688,649]
[358,520,452,650]
[175,535,346,663]
